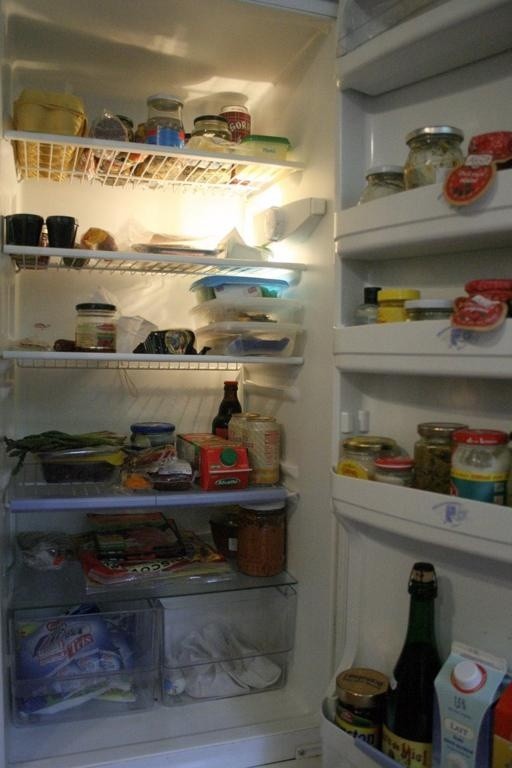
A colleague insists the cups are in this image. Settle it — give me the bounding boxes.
[46,216,79,249]
[6,213,44,245]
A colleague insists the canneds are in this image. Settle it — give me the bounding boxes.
[73,303,117,353]
[355,123,464,210]
[114,114,232,143]
[243,415,281,486]
[376,278,512,323]
[207,502,287,577]
[229,412,260,444]
[130,421,175,461]
[338,422,512,507]
[335,666,390,749]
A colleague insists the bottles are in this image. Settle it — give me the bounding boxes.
[380,558,443,768]
[357,125,466,205]
[211,379,244,438]
[352,286,383,324]
[404,300,454,321]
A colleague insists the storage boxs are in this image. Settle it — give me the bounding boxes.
[8,600,165,726]
[159,585,301,708]
